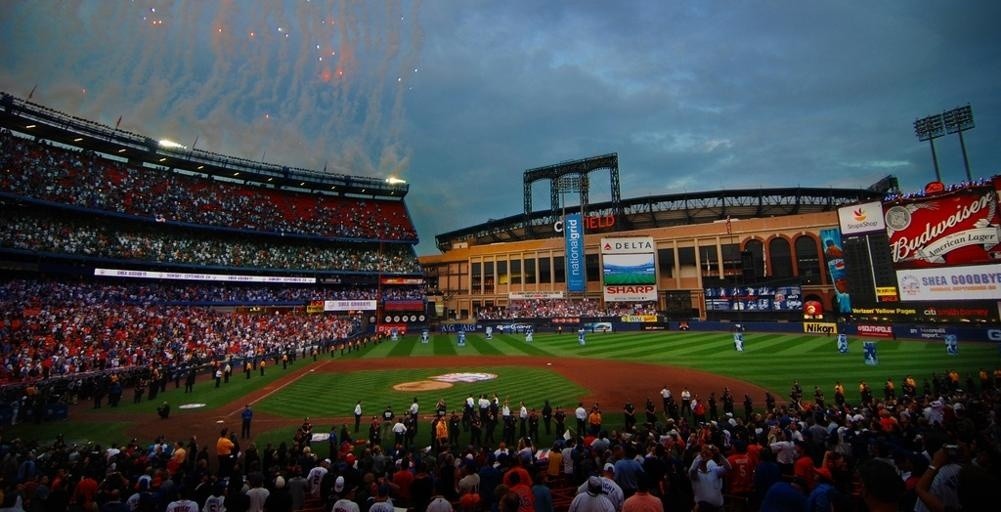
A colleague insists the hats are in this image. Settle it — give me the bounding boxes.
[603,462,616,473]
[725,412,733,417]
[710,421,717,426]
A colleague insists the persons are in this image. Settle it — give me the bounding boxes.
[0,129,428,362]
[471,294,662,337]
[0,362,1000,511]
